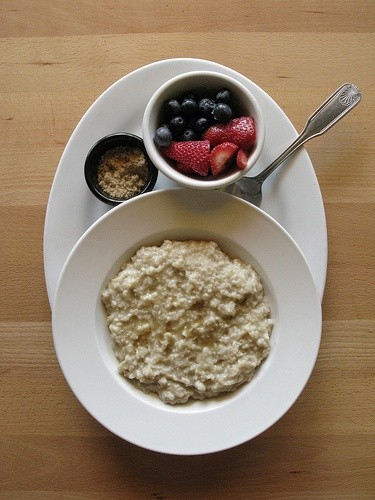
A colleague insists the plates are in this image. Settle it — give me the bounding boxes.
[42,58,327,304]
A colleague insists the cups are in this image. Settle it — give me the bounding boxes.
[83,131,158,206]
[142,70,266,188]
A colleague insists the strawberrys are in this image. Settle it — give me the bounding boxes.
[161,115,256,176]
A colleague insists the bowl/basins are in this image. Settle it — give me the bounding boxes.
[50,188,323,455]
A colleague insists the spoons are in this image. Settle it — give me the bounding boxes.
[217,83,363,208]
